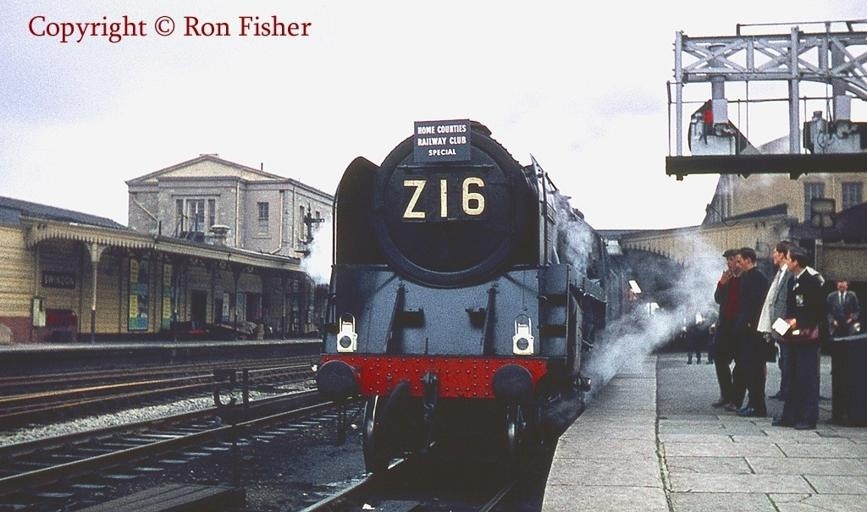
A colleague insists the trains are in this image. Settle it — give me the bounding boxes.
[317,119,631,500]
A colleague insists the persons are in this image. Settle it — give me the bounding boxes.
[706,247,746,412]
[731,248,768,418]
[766,238,796,428]
[824,275,863,337]
[699,301,718,365]
[681,305,702,364]
[770,243,821,431]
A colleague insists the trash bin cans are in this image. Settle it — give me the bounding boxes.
[830,334,867,427]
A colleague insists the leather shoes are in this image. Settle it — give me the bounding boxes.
[772,415,817,430]
[711,398,767,417]
